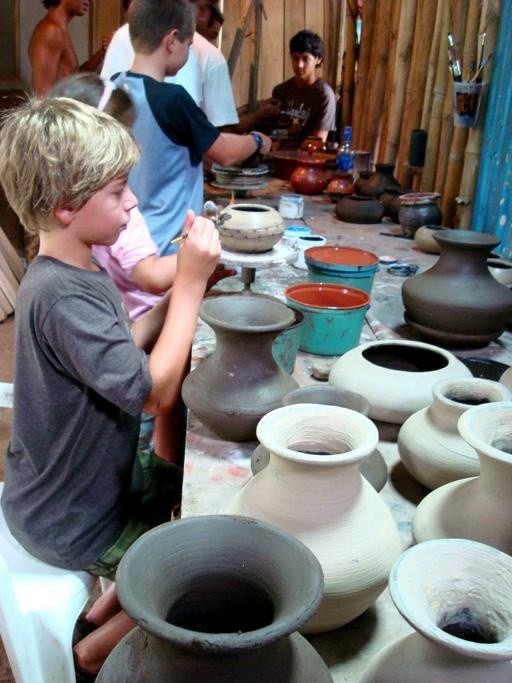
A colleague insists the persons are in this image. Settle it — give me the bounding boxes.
[96,22,240,130]
[107,1,272,258]
[271,30,337,146]
[27,0,115,97]
[195,2,280,133]
[0,94,222,681]
[47,74,180,324]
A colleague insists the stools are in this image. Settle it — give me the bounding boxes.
[0,482,98,683]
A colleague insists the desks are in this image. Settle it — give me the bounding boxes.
[181,171,512,683]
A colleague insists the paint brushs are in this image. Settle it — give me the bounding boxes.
[467,62,475,82]
[477,32,486,83]
[171,217,218,244]
[447,32,462,83]
[468,50,495,82]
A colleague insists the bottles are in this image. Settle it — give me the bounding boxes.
[335,126,355,174]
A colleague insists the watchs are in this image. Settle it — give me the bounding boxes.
[251,132,263,150]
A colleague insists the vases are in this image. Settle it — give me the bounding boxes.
[181,293,305,443]
[250,383,388,495]
[357,538,512,683]
[402,228,512,351]
[223,402,401,636]
[397,379,512,491]
[94,513,335,683]
[411,402,512,554]
